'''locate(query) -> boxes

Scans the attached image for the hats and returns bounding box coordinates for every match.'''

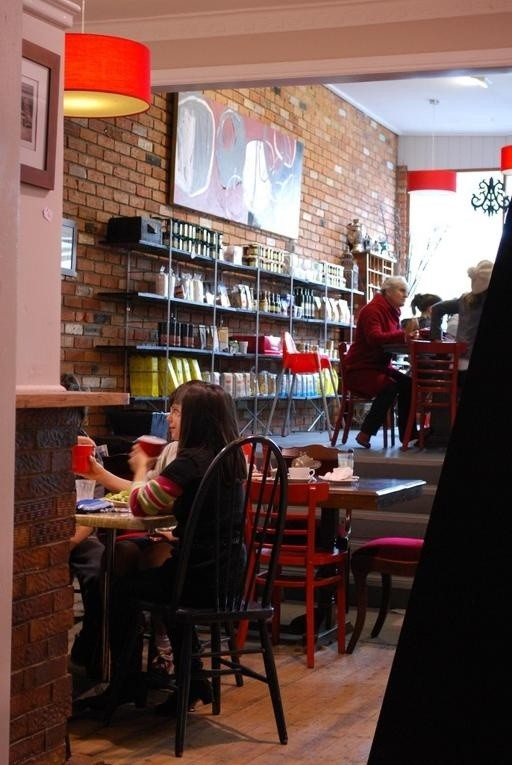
[467,260,493,294]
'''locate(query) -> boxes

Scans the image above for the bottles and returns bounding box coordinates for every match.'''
[155,266,168,296]
[157,320,167,346]
[181,324,189,347]
[303,289,308,316]
[163,217,224,261]
[259,290,267,311]
[310,289,315,320]
[242,242,287,274]
[265,290,282,314]
[176,323,181,346]
[168,322,176,347]
[295,287,305,319]
[187,325,195,347]
[168,269,175,297]
[305,289,311,320]
[291,254,351,288]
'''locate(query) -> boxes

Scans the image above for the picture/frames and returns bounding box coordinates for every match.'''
[20,39,61,190]
[61,216,77,276]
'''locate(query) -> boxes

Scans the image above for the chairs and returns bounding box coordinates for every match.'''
[116,434,289,758]
[233,475,349,669]
[403,341,461,451]
[242,436,272,475]
[346,537,425,654]
[329,342,396,449]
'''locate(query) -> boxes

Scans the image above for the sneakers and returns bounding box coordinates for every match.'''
[401,431,419,442]
[355,433,370,448]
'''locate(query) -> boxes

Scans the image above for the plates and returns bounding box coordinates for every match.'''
[102,497,132,508]
[319,475,358,483]
[286,479,313,483]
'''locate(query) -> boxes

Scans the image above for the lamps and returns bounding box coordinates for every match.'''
[407,99,456,194]
[501,145,511,172]
[64,0,150,118]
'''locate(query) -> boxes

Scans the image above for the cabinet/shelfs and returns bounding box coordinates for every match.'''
[93,214,365,438]
[343,251,395,342]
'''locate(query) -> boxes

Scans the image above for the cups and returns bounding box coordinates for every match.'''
[76,480,96,503]
[338,453,354,470]
[334,468,353,480]
[71,445,95,478]
[136,435,167,470]
[288,468,315,479]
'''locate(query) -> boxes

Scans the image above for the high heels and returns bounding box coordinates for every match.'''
[156,677,213,714]
[79,679,147,710]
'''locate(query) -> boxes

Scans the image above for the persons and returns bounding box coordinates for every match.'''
[429,260,493,384]
[76,379,204,674]
[446,297,470,384]
[339,275,431,449]
[402,293,442,390]
[73,383,248,711]
[60,372,109,670]
